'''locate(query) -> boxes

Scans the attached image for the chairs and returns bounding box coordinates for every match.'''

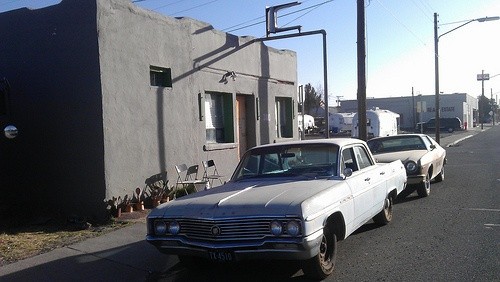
[185,165,208,192]
[173,164,194,199]
[202,161,224,187]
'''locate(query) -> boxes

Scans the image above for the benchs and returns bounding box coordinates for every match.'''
[283,163,336,175]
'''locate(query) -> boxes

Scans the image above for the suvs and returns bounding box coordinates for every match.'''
[416,116,463,133]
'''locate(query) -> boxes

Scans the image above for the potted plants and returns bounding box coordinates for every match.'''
[126,203,133,213]
[152,192,161,208]
[137,200,144,212]
[115,205,121,217]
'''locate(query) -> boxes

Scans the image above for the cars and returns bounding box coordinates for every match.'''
[146,137,408,282]
[344,133,448,198]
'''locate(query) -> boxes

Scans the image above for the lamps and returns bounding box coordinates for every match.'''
[222,71,237,83]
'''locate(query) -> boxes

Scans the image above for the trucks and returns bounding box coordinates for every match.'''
[297,112,315,135]
[350,106,400,141]
[329,111,356,134]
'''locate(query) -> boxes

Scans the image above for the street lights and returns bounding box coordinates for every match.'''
[433,16,500,146]
[481,73,500,130]
[418,94,423,134]
